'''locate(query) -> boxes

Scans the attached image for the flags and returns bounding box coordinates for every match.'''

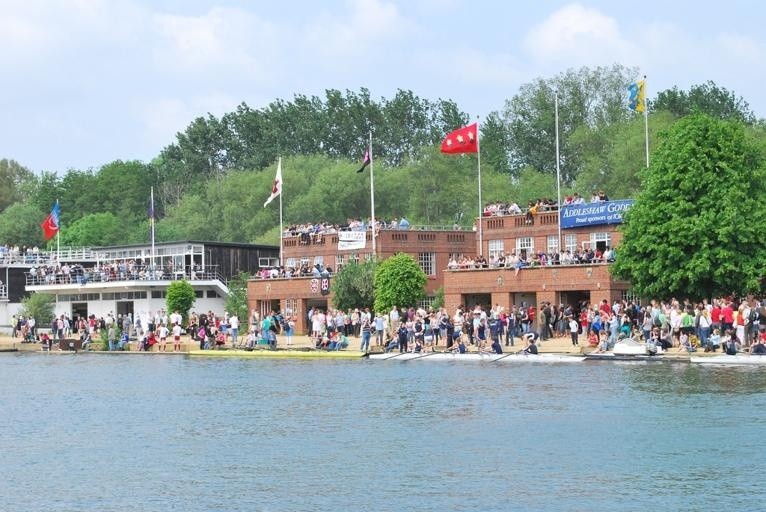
[41,204,60,241]
[441,122,479,157]
[261,162,284,207]
[355,143,373,175]
[147,199,157,241]
[628,76,647,114]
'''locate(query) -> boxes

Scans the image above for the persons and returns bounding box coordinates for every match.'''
[30,261,200,284]
[453,222,463,231]
[562,190,609,206]
[256,264,333,279]
[305,299,766,355]
[1,244,39,266]
[482,199,522,217]
[285,216,410,244]
[11,312,294,354]
[447,245,617,272]
[527,198,558,225]
